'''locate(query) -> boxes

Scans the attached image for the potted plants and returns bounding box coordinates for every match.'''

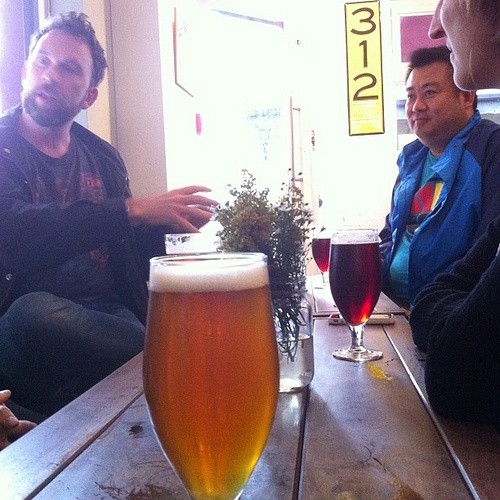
[213,169,327,394]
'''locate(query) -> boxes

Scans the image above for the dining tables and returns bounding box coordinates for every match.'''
[0,273,500,500]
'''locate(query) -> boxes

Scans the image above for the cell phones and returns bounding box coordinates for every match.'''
[328,313,395,325]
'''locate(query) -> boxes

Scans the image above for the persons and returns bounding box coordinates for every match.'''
[379,44,500,308]
[408,0,499,444]
[0,10,217,398]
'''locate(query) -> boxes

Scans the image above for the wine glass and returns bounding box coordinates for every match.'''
[140,251,280,500]
[328,229,383,362]
[311,231,331,289]
